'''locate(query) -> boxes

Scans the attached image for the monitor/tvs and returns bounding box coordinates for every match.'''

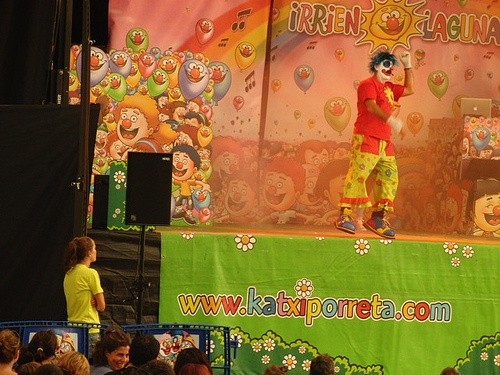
[460,97,492,120]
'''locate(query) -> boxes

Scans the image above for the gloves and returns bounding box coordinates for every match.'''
[398,52,412,68]
[387,115,403,134]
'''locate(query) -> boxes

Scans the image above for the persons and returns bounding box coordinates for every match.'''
[0,329,90,375]
[63,237,106,363]
[90,329,175,375]
[440,367,459,375]
[334,51,415,238]
[264,354,335,375]
[175,348,212,375]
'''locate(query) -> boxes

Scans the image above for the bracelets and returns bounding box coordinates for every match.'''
[404,68,410,70]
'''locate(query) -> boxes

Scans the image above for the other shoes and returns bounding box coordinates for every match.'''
[336,208,355,234]
[362,211,395,239]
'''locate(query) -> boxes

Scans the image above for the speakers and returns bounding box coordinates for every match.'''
[92,175,125,229]
[125,151,172,226]
